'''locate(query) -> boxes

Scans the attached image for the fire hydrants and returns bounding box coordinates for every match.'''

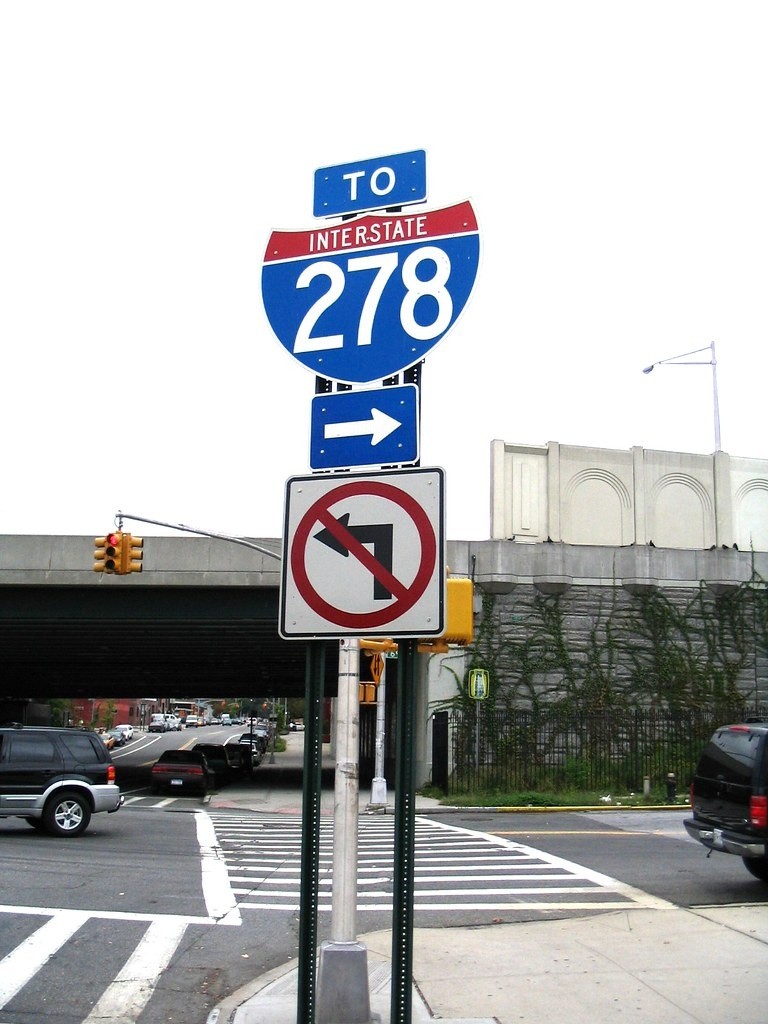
[665,772,677,804]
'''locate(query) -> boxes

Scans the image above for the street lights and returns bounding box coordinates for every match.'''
[644,339,724,453]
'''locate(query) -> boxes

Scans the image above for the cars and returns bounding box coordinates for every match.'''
[209,718,218,724]
[217,713,305,760]
[192,742,230,787]
[226,742,250,779]
[98,733,114,750]
[151,749,215,794]
[198,717,206,726]
[107,729,127,746]
[115,724,134,741]
[148,713,182,732]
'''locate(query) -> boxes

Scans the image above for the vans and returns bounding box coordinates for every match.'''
[185,715,199,728]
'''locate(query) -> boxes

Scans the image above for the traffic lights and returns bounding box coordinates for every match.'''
[105,530,124,574]
[94,535,105,573]
[125,533,144,574]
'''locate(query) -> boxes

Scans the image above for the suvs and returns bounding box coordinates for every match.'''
[682,722,768,885]
[0,722,127,839]
